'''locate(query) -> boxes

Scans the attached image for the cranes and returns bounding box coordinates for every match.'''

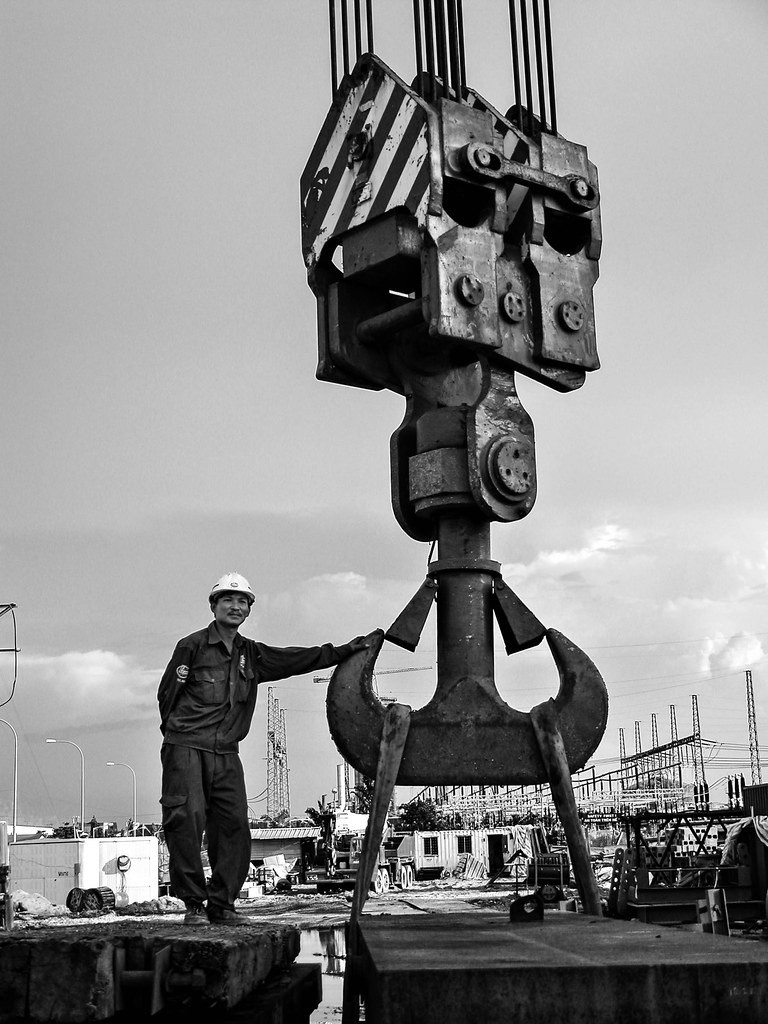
[312,661,434,815]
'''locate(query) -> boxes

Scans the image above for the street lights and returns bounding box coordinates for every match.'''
[105,761,137,837]
[44,738,85,836]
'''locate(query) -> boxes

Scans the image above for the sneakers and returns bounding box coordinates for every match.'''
[206,904,251,927]
[185,904,210,925]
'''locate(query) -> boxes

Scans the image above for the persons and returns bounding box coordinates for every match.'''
[149,567,382,929]
[85,815,98,838]
[106,818,133,837]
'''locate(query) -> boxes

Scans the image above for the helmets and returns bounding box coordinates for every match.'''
[208,572,255,607]
